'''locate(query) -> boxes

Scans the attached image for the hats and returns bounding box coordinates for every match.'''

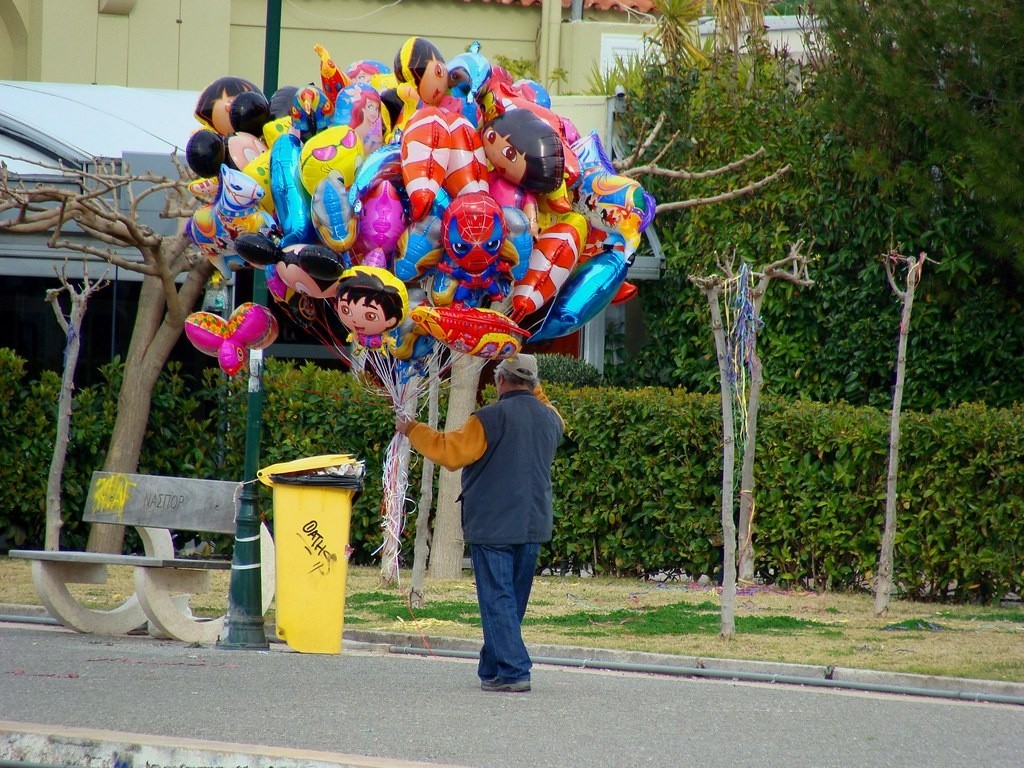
[499,353,538,381]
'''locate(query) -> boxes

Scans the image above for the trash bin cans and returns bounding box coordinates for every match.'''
[256,454,367,656]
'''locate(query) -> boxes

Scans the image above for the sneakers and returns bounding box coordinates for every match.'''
[481,674,531,692]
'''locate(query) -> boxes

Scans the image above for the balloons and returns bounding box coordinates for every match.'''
[172,38,652,373]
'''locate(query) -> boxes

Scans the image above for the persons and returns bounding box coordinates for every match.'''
[395,353,565,690]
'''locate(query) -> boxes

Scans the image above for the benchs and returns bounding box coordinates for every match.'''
[9,473,276,651]
